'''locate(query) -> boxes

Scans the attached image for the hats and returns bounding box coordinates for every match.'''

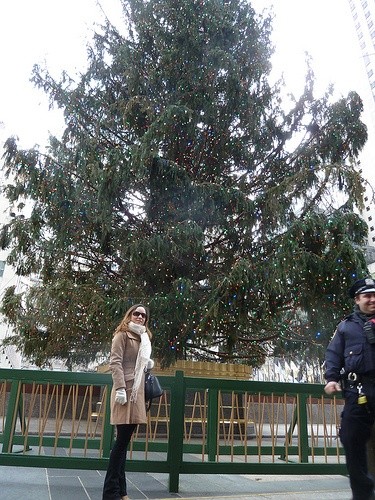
[350,278,375,299]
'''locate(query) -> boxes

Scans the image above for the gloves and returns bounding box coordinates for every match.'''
[115,387,127,405]
[147,359,154,369]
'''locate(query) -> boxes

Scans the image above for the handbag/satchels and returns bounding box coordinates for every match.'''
[144,374,164,401]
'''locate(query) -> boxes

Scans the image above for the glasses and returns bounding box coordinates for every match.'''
[133,311,146,318]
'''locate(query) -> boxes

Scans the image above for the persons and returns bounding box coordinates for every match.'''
[102,304,154,500]
[324,279,375,500]
